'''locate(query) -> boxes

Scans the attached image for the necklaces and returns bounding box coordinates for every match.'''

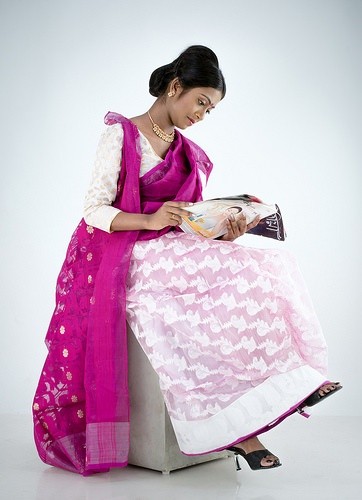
[147,110,177,143]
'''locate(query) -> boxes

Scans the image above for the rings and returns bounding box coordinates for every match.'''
[170,214,176,219]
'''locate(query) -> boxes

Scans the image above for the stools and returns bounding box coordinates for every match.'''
[124,318,237,474]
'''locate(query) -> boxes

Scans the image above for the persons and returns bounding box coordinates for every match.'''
[190,206,242,236]
[62,43,344,472]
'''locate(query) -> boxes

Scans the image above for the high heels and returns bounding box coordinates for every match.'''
[296,382,343,418]
[226,447,282,470]
